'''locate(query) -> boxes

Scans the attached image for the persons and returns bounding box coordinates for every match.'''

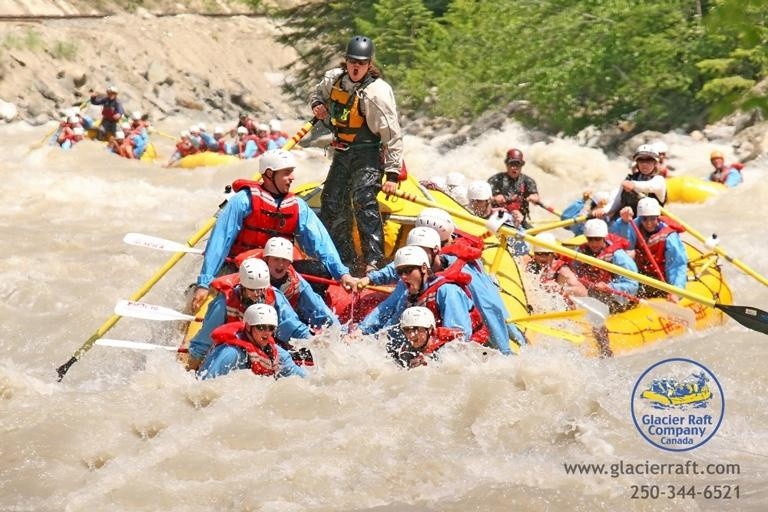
[51,86,153,160]
[187,137,690,379]
[174,111,289,158]
[306,35,403,273]
[705,150,745,187]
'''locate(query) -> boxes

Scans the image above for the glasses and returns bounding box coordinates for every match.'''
[403,326,418,333]
[587,237,603,241]
[349,58,368,65]
[396,266,415,275]
[255,325,275,331]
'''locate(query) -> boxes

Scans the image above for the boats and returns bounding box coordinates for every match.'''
[509,231,731,363]
[173,168,529,381]
[655,173,732,205]
[166,148,250,171]
[78,113,155,167]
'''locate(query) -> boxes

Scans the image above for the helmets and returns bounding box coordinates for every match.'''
[400,306,437,331]
[239,111,250,119]
[239,236,293,326]
[58,107,83,136]
[593,190,610,207]
[632,139,668,163]
[346,35,374,60]
[585,218,608,238]
[258,148,298,173]
[107,87,118,94]
[711,150,724,160]
[533,232,555,253]
[446,172,465,187]
[237,119,281,135]
[468,181,492,201]
[637,197,661,217]
[394,207,454,269]
[505,149,525,166]
[115,111,142,139]
[180,123,225,137]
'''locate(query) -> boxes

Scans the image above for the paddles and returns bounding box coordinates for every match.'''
[370,182,768,336]
[115,299,206,322]
[125,233,354,292]
[577,277,697,327]
[56,117,320,389]
[93,338,193,353]
[507,295,612,326]
